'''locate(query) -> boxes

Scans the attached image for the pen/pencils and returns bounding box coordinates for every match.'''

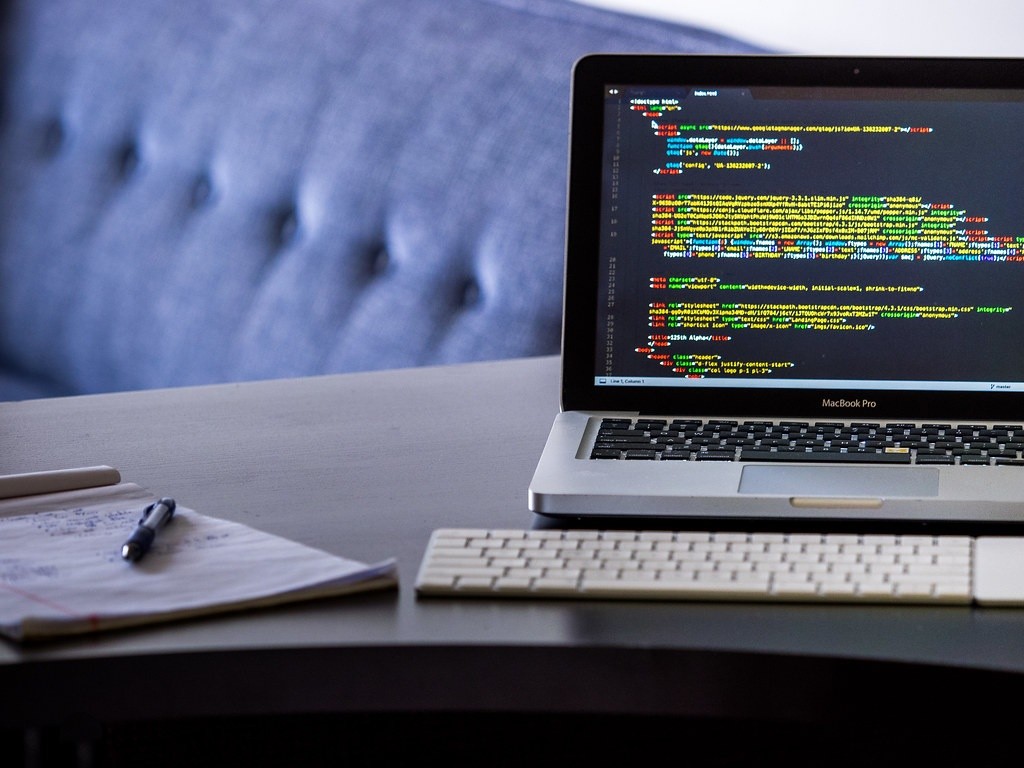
[120,497,179,568]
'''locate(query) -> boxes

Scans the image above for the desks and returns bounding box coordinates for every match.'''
[0,353,1022,766]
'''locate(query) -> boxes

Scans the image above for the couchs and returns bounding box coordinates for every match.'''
[0,1,788,399]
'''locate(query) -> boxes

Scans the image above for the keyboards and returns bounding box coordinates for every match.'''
[413,528,972,600]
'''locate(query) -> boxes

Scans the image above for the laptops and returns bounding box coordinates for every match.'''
[524,53,1024,522]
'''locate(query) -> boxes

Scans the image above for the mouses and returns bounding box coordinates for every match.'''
[977,539,1024,604]
